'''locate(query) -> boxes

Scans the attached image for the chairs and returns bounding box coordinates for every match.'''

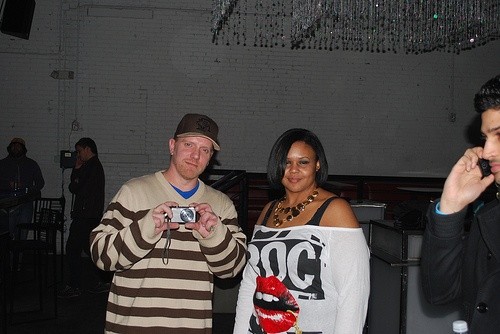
[7,196,65,325]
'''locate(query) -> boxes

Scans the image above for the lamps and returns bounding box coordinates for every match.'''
[207,0,500,54]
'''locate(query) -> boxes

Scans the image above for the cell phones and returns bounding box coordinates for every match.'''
[479,159,491,176]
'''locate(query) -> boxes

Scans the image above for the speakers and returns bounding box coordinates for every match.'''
[0,0,36,41]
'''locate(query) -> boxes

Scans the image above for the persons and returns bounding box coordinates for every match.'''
[89,113,248,334]
[418,73,499,333]
[231,127,371,334]
[57,137,111,298]
[0,137,45,271]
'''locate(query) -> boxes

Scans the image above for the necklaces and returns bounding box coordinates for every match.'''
[273,189,319,227]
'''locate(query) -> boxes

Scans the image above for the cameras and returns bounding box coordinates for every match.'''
[164,207,196,223]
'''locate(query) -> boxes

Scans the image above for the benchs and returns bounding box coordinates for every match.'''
[196,168,500,231]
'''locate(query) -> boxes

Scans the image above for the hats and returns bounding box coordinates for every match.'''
[174,113,220,151]
[7,138,27,153]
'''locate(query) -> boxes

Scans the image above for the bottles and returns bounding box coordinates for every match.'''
[453,320,470,334]
